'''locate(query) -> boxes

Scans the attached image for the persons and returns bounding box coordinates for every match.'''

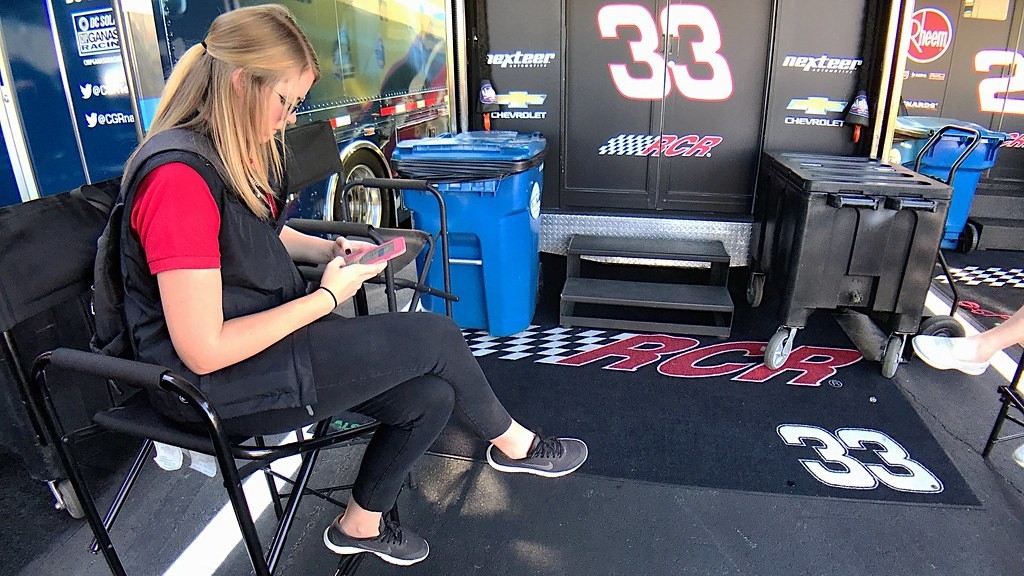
[117,4,587,565]
[911,305,1024,469]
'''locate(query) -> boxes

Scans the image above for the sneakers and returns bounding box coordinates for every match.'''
[323,514,430,567]
[486,432,588,478]
[912,335,990,375]
[1013,444,1024,467]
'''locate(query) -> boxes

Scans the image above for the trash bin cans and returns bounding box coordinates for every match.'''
[758,146,952,331]
[389,131,549,337]
[889,115,1006,252]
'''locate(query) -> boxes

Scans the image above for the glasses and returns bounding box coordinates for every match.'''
[254,77,306,115]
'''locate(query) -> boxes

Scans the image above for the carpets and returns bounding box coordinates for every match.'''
[301,306,988,515]
[930,247,1024,368]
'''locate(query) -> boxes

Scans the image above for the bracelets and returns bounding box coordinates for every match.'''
[319,287,337,309]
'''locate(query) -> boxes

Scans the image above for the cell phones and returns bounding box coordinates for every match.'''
[340,237,406,269]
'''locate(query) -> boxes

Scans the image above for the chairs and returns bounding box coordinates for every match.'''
[0,173,403,576]
[268,117,461,321]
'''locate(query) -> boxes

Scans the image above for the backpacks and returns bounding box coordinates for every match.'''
[70,129,234,398]
